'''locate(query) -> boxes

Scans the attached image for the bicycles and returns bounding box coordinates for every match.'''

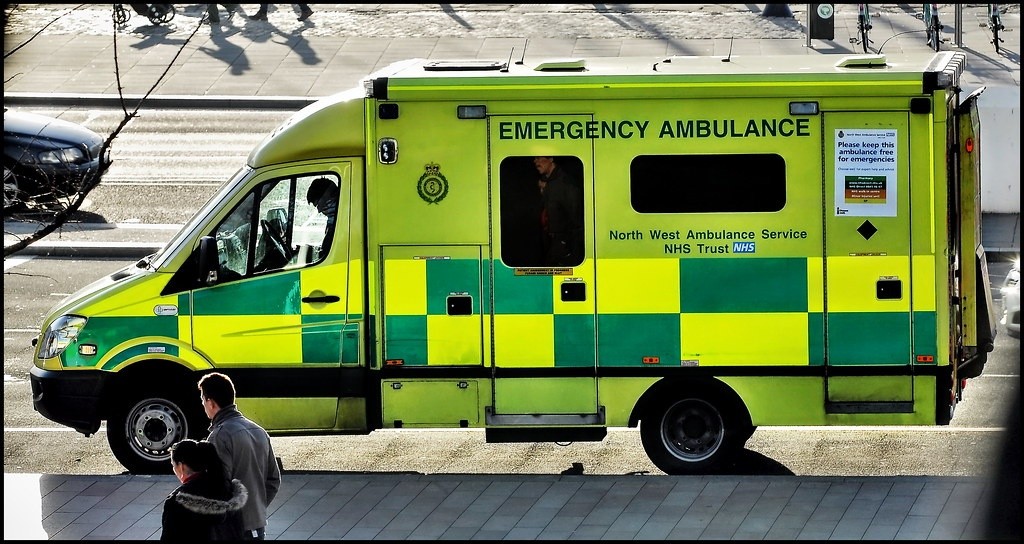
[849,4,880,52]
[915,3,944,51]
[985,4,1005,53]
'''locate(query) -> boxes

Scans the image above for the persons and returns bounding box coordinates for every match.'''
[307,177,338,257]
[159,439,247,539]
[197,373,281,539]
[501,156,585,266]
[199,4,315,24]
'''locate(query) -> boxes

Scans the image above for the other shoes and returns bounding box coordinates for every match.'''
[249,10,268,21]
[299,8,314,21]
[203,17,219,25]
[227,7,237,22]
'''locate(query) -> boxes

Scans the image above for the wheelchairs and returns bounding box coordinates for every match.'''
[111,3,175,25]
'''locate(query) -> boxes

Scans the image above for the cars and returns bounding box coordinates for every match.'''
[4,107,110,216]
[1002,260,1020,340]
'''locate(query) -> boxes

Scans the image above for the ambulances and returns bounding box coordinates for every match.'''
[29,52,998,475]
